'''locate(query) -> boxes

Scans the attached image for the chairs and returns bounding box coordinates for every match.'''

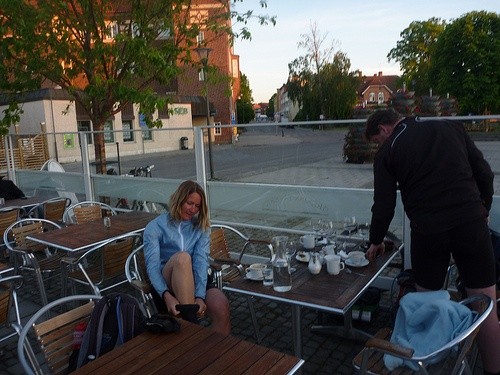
[0,195,493,375]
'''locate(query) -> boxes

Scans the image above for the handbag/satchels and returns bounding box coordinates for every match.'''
[383,289,480,371]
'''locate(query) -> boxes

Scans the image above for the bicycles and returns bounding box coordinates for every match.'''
[110,165,169,214]
[339,224,458,290]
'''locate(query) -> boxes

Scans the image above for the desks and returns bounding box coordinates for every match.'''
[25,210,161,308]
[72,316,306,375]
[221,234,405,375]
[1,193,63,228]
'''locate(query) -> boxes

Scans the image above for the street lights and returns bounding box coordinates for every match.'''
[195,46,218,181]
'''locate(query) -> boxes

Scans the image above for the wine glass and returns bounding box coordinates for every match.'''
[313,225,322,246]
[358,223,369,250]
[343,217,357,247]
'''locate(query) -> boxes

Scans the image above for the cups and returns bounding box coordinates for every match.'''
[318,218,335,236]
[325,255,345,275]
[262,268,274,287]
[348,251,365,264]
[299,234,315,249]
[245,263,267,277]
[104,217,111,227]
[336,232,346,255]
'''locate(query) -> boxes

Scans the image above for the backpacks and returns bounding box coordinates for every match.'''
[74,292,147,371]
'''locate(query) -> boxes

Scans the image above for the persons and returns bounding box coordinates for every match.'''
[143,180,230,335]
[365,109,500,375]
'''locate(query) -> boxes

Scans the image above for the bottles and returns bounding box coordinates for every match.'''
[308,256,322,275]
[272,236,293,293]
[72,322,87,349]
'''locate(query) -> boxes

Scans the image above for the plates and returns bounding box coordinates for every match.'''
[344,259,369,267]
[295,254,310,262]
[245,272,263,280]
